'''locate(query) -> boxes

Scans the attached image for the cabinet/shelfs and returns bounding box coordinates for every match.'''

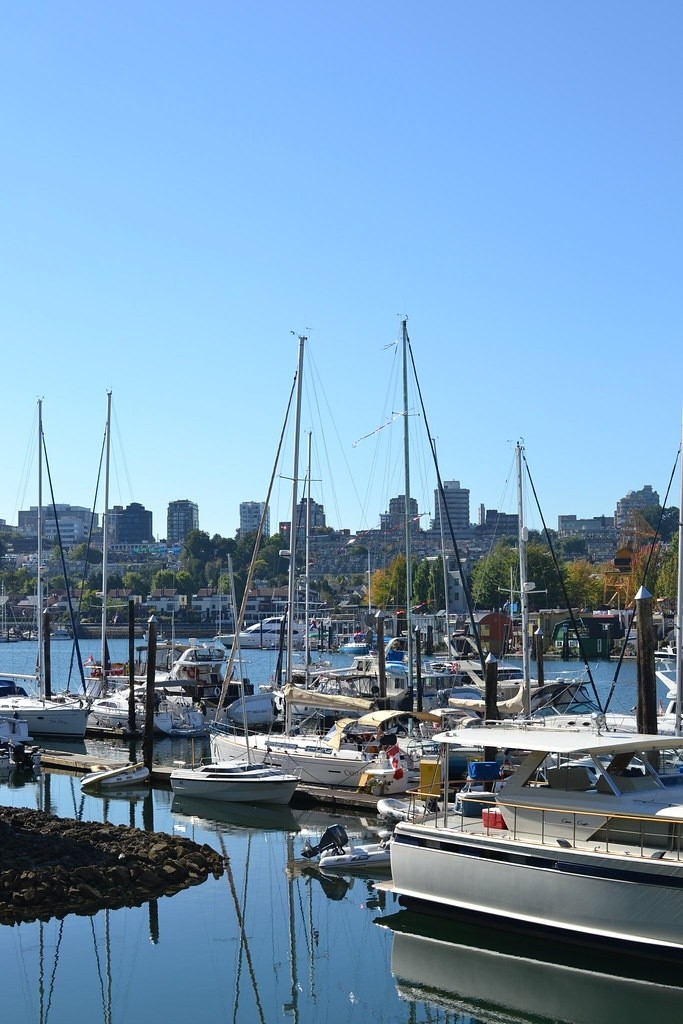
[419,760,442,800]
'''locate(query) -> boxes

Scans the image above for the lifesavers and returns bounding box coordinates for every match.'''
[213,688,221,697]
[451,663,461,673]
[186,667,197,677]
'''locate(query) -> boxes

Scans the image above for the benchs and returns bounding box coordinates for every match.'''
[545,768,595,791]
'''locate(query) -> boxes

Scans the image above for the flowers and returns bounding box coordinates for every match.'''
[367,777,392,791]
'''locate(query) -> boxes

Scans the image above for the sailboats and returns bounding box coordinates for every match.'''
[0,312,683,955]
[26,738,88,820]
[168,794,304,1024]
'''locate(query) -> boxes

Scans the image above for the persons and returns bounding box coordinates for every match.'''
[154,693,207,717]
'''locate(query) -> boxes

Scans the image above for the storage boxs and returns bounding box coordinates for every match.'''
[455,791,498,816]
[482,808,506,829]
[468,762,499,780]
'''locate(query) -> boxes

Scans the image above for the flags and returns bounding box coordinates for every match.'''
[309,617,317,632]
[388,745,403,779]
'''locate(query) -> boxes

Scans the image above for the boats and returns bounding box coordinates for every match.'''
[319,867,390,883]
[372,908,683,1024]
[80,784,150,798]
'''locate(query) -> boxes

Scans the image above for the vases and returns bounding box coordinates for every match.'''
[371,786,383,796]
